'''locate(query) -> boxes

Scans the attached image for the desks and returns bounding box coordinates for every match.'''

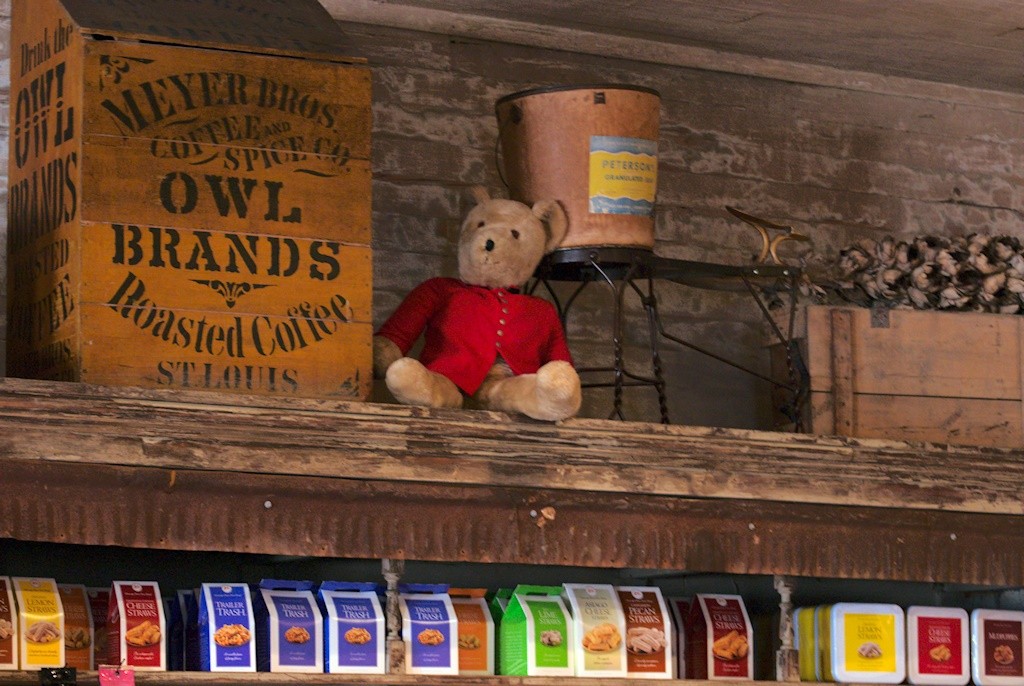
[522,250,805,431]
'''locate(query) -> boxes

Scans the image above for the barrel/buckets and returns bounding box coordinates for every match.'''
[492,83,659,251]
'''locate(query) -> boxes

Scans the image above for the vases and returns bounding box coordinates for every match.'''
[806,305,1024,447]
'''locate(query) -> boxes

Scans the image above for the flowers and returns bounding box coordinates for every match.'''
[821,230,1024,310]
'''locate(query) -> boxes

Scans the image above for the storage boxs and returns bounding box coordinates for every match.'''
[10,0,373,399]
[793,604,1024,686]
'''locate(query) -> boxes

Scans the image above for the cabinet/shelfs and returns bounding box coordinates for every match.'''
[0,374,1024,686]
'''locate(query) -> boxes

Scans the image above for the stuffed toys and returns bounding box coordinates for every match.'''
[372,184,582,422]
